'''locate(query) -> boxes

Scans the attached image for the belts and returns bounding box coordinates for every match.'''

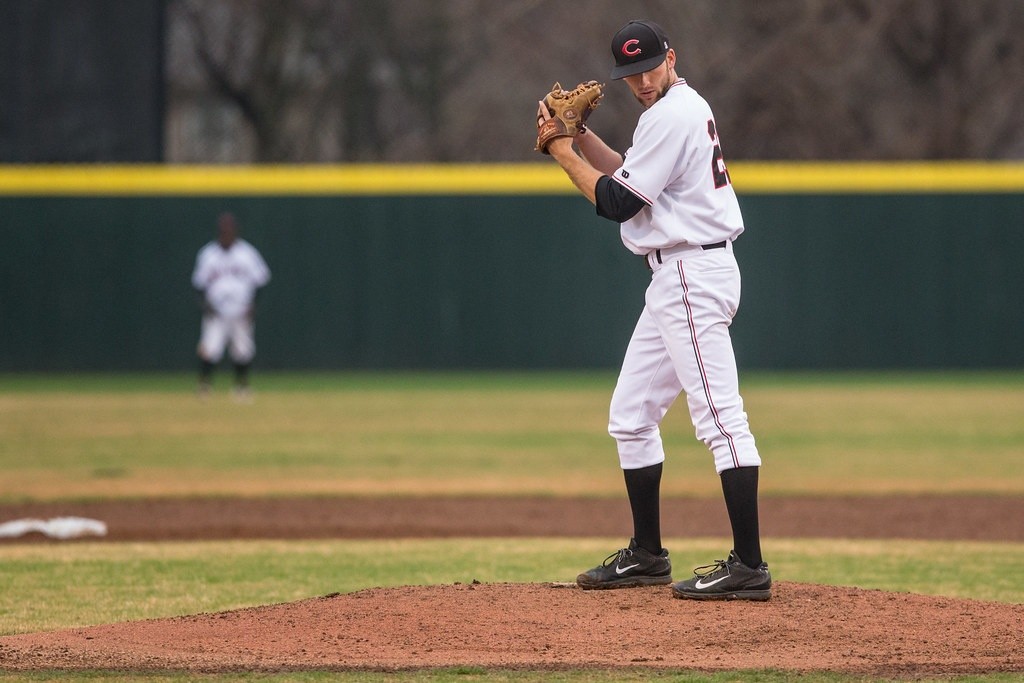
[644,240,726,270]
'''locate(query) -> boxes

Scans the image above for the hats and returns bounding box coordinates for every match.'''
[609,20,671,81]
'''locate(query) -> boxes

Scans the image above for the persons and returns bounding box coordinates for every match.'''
[192,213,271,383]
[534,20,773,601]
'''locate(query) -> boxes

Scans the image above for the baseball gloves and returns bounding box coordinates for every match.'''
[534,79,606,155]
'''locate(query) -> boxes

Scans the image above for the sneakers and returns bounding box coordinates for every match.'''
[672,550,771,601]
[576,537,674,590]
